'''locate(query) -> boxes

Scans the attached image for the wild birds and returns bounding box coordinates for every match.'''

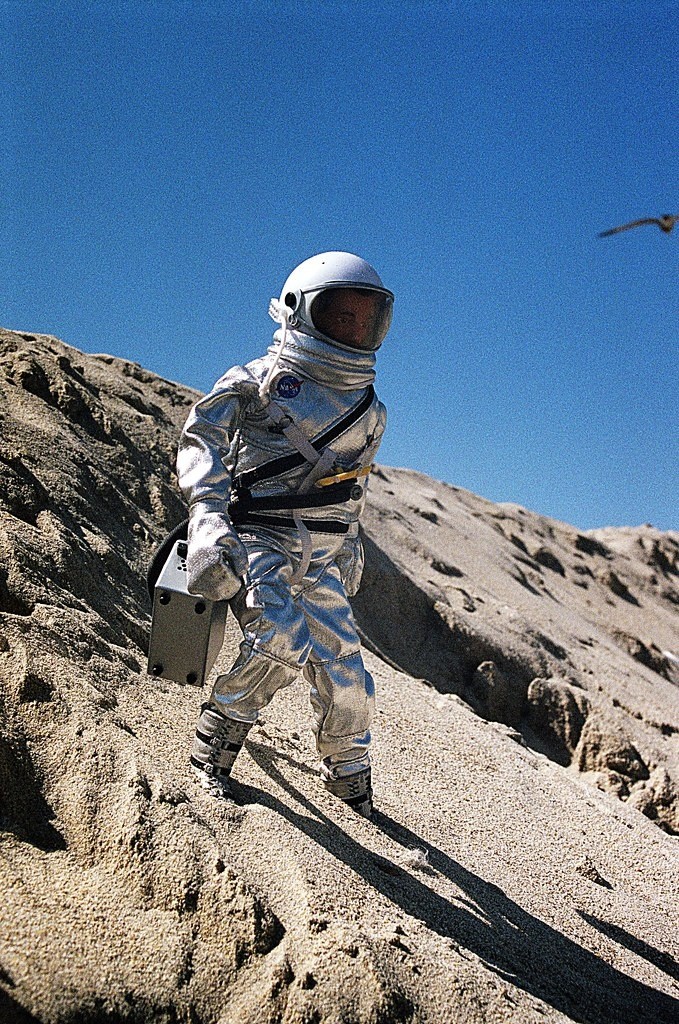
[599,214,679,238]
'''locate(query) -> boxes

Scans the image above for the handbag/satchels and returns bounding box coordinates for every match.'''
[145,518,229,688]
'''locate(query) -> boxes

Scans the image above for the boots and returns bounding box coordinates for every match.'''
[319,765,372,822]
[191,702,254,803]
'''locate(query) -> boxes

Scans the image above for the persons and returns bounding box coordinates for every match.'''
[177,249,396,816]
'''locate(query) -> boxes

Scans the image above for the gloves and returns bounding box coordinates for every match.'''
[185,496,249,603]
[335,521,364,598]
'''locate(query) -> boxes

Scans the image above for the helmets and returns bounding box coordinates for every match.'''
[269,250,396,356]
